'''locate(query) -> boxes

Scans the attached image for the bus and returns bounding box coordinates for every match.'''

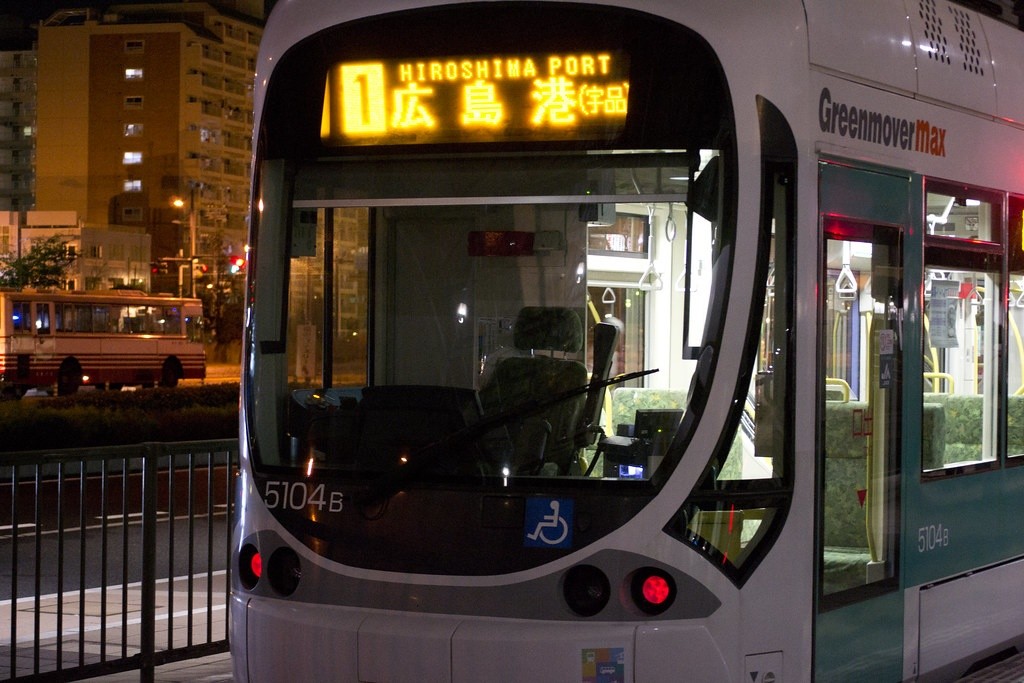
[0,289,209,402]
[226,0,1023,683]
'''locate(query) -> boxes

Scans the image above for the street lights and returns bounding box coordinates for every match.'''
[170,182,203,300]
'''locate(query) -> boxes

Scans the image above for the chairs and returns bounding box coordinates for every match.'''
[437,303,1024,594]
